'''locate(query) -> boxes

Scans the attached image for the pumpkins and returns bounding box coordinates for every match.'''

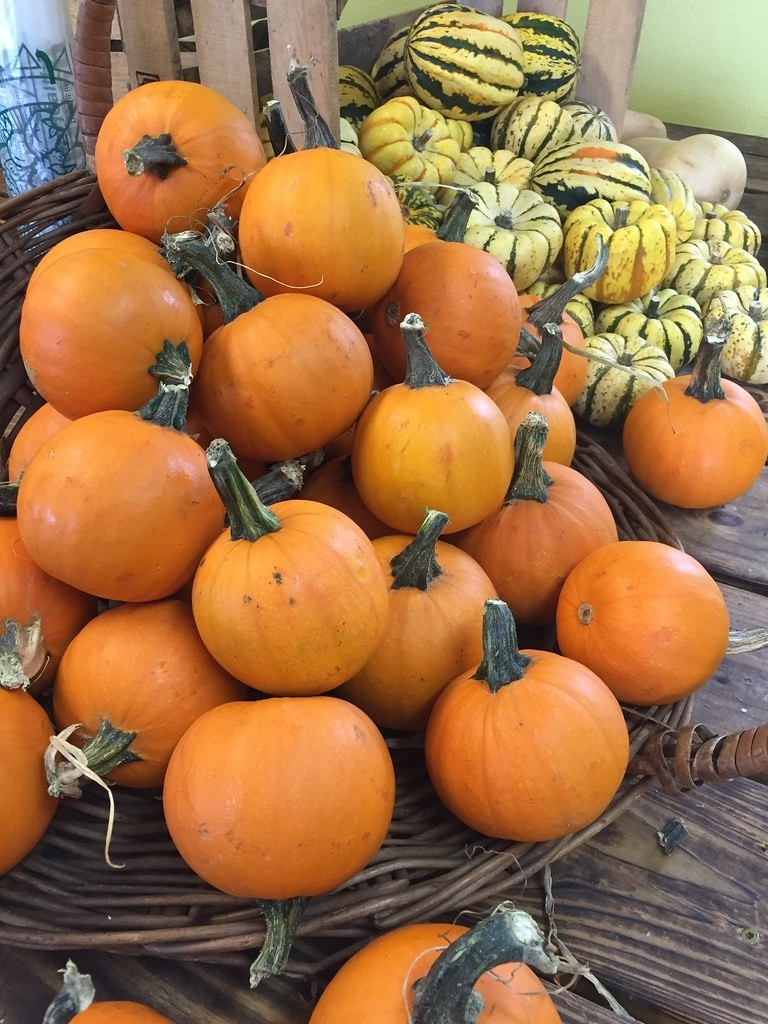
[0,4,768,1024]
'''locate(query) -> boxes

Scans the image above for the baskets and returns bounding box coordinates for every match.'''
[0,0,768,976]
